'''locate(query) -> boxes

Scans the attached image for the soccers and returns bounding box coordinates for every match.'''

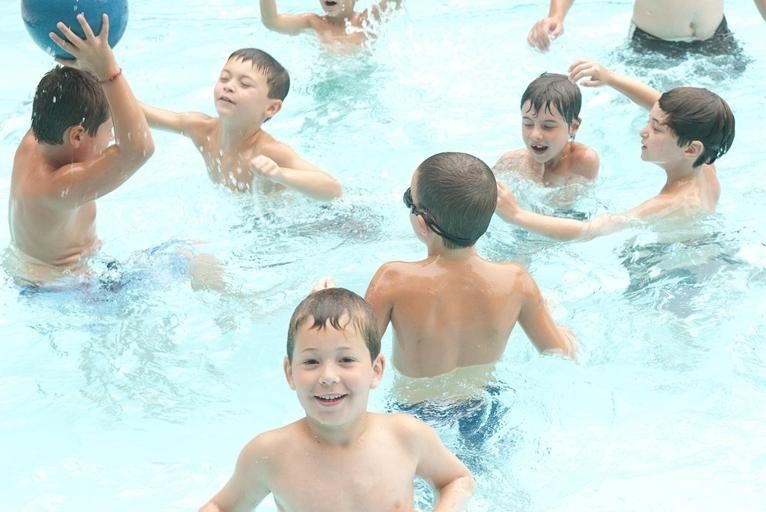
[21,0,128,61]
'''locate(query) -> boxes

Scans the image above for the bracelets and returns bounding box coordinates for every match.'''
[99,68,122,83]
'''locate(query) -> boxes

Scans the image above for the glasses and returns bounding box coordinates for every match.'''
[403,188,472,246]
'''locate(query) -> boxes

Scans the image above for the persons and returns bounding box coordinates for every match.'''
[260,0,403,57]
[310,152,579,473]
[4,14,221,309]
[527,0,766,86]
[495,59,735,243]
[198,288,476,512]
[491,73,599,208]
[137,48,342,202]
